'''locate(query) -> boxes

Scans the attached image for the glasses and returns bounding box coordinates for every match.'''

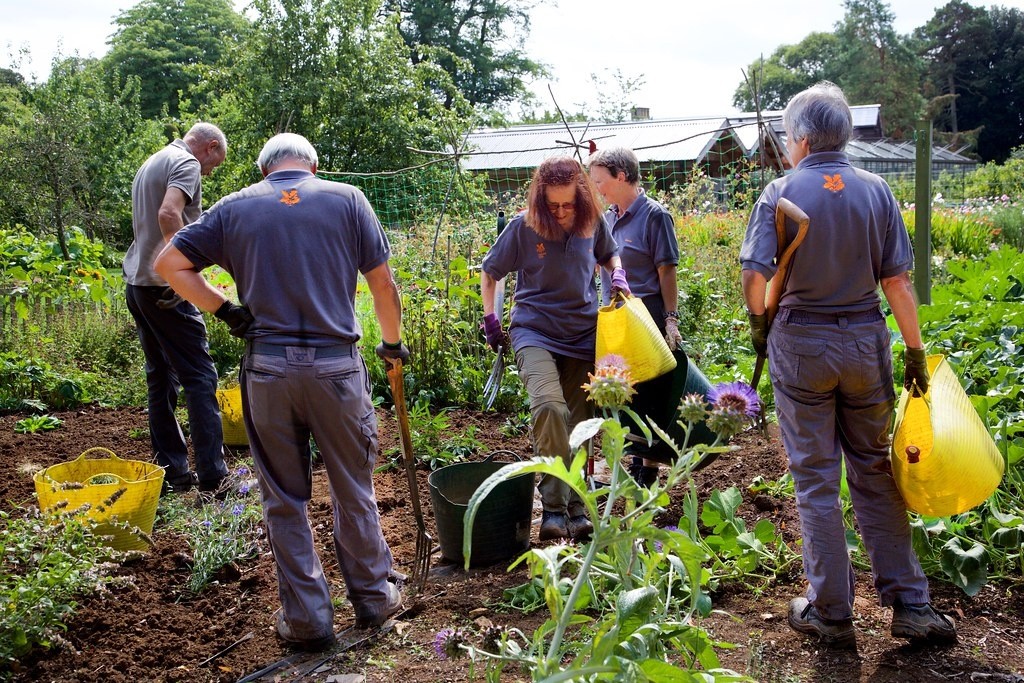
[543,198,577,212]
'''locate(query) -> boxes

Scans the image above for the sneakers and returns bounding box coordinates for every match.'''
[628,464,657,488]
[538,510,570,538]
[786,596,855,640]
[892,601,956,637]
[567,515,593,537]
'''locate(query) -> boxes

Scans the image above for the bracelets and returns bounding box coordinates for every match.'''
[664,312,679,322]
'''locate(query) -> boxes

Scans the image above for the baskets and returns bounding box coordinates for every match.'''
[427,450,536,569]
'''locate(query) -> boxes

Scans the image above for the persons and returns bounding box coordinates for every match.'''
[481,155,631,540]
[123,122,250,505]
[154,132,409,642]
[739,84,955,645]
[587,147,682,490]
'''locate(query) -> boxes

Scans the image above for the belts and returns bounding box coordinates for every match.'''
[251,342,352,358]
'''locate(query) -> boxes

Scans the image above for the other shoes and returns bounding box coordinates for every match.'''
[199,486,234,502]
[278,613,336,646]
[161,470,198,493]
[358,580,402,627]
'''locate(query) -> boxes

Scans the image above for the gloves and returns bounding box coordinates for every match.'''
[903,344,930,398]
[482,312,509,353]
[663,316,682,350]
[375,339,409,370]
[214,302,254,337]
[156,284,186,309]
[748,309,769,358]
[608,268,632,301]
